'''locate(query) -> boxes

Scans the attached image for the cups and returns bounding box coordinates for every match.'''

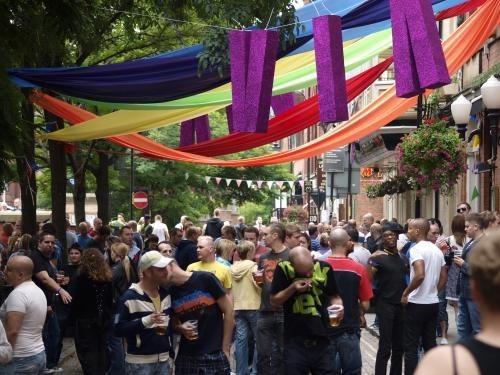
[156,313,169,336]
[56,271,64,283]
[254,271,263,283]
[327,305,344,327]
[185,320,198,340]
[454,251,462,259]
[437,235,450,254]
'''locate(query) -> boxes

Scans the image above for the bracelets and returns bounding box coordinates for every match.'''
[56,287,63,293]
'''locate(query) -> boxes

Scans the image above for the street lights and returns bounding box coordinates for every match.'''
[481,75,499,211]
[451,94,471,208]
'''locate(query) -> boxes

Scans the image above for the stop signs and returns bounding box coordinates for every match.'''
[132,192,149,210]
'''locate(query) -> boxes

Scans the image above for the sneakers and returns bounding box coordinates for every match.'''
[440,338,448,345]
[369,323,380,336]
[47,367,63,373]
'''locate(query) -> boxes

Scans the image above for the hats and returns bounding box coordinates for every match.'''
[140,250,174,273]
[382,225,405,234]
[144,215,150,219]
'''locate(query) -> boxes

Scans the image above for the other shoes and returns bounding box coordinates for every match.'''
[438,332,443,337]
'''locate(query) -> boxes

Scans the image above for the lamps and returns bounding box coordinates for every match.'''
[481,74,500,146]
[449,94,484,151]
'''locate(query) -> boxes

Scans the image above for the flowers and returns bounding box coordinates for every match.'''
[395,115,476,200]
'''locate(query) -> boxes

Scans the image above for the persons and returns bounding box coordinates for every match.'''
[0,197,500,375]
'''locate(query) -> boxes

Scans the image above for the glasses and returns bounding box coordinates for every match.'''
[429,220,439,225]
[457,208,467,212]
